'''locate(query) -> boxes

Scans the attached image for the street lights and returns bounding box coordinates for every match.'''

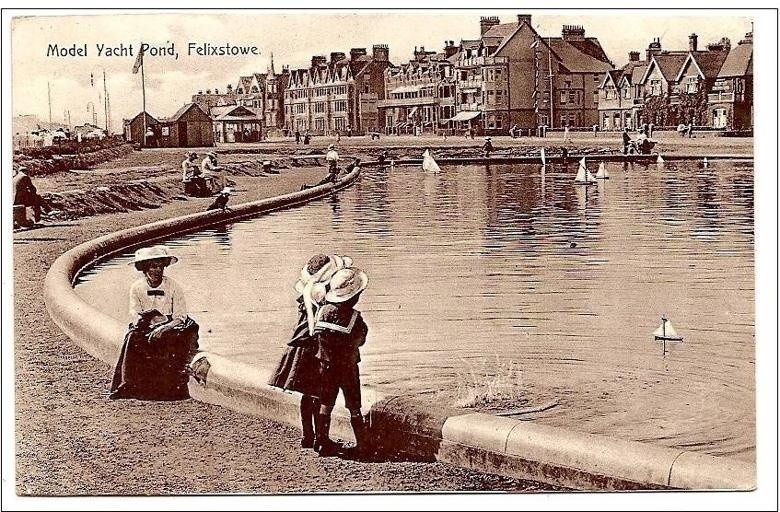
[536,23,555,135]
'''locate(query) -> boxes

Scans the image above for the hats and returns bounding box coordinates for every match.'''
[127,246,178,265]
[295,255,368,305]
[19,166,28,171]
[221,187,231,195]
[327,144,335,151]
[207,151,217,160]
[184,151,199,160]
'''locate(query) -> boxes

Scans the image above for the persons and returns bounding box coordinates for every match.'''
[15,128,70,147]
[108,244,199,402]
[13,166,60,225]
[635,129,648,146]
[225,128,235,142]
[677,120,692,137]
[342,158,361,174]
[564,124,574,143]
[375,153,385,167]
[269,254,368,454]
[442,131,448,142]
[465,125,481,139]
[182,151,224,196]
[300,168,342,191]
[638,121,655,138]
[347,126,352,137]
[304,129,311,144]
[593,123,599,137]
[623,128,631,154]
[336,132,340,143]
[145,127,154,148]
[206,187,232,210]
[295,129,300,144]
[326,144,339,173]
[242,127,249,142]
[482,137,494,157]
[509,124,518,138]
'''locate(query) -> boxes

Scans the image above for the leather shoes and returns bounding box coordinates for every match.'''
[48,210,60,216]
[301,434,374,458]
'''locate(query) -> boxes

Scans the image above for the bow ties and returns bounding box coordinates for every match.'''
[147,290,164,296]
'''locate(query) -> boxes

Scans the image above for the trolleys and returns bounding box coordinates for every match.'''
[627,138,659,157]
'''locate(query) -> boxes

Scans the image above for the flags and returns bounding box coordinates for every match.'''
[132,45,142,74]
